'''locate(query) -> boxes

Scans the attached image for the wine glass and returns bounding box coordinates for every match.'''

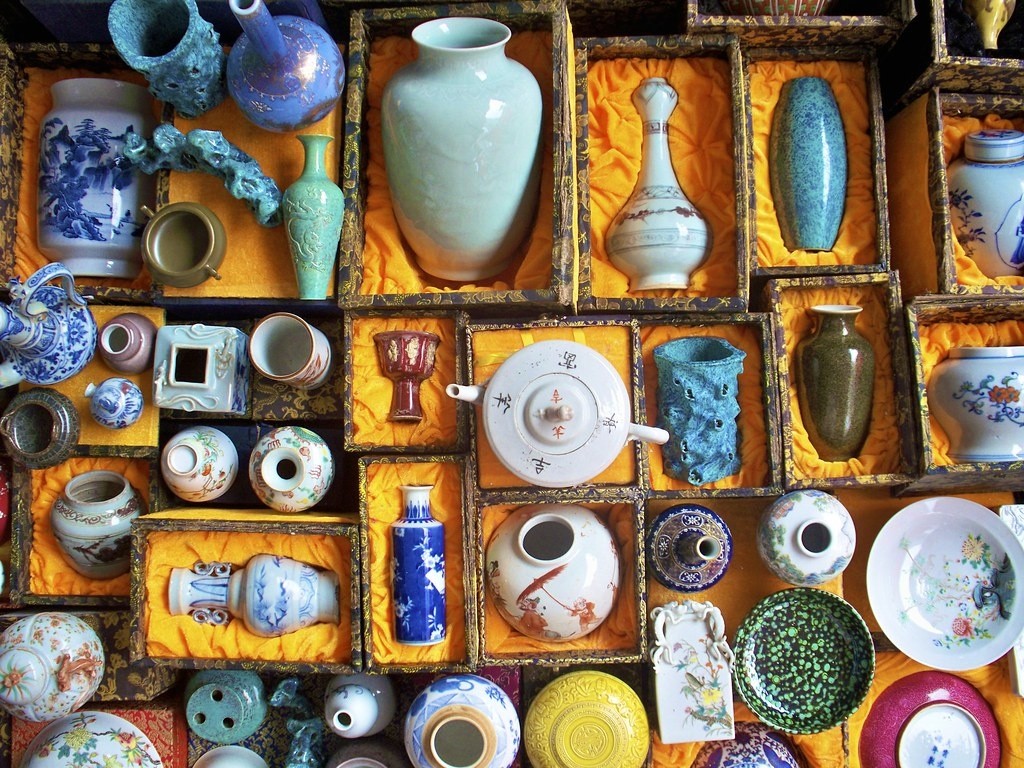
[373,331,440,421]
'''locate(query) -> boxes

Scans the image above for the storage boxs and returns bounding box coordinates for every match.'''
[0,0,1024,768]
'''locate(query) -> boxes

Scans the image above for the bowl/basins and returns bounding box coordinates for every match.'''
[141,202,227,287]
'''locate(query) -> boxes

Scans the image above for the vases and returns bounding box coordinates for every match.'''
[604,78,712,292]
[404,674,521,768]
[168,554,340,638]
[485,503,622,643]
[389,484,446,646]
[644,503,733,595]
[37,78,150,280]
[383,16,544,281]
[755,489,857,587]
[107,0,230,118]
[152,324,250,415]
[227,0,345,134]
[248,312,337,391]
[282,134,345,301]
[653,335,747,485]
[48,470,145,581]
[98,312,156,374]
[794,303,875,462]
[767,75,849,253]
[324,666,396,739]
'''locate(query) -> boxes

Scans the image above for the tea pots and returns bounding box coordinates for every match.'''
[446,339,669,489]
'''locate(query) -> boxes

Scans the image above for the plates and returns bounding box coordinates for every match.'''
[866,496,1024,671]
[732,587,875,735]
[858,670,1003,768]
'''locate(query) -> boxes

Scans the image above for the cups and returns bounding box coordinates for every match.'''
[249,312,338,390]
